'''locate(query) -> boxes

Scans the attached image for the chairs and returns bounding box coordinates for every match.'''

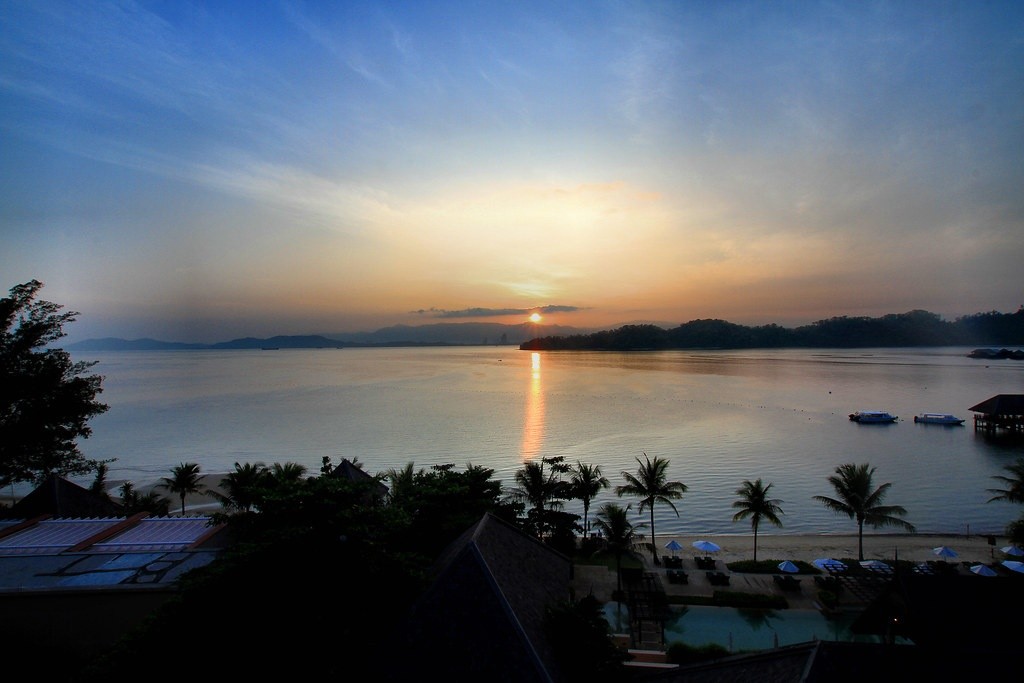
[785,576,801,591]
[672,556,683,569]
[677,571,688,584]
[826,576,840,591]
[667,570,680,583]
[694,557,707,569]
[707,572,722,585]
[718,572,731,585]
[705,557,716,570]
[814,576,832,590]
[773,576,792,591]
[663,556,674,568]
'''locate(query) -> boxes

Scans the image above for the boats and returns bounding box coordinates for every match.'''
[848,410,898,423]
[914,413,965,425]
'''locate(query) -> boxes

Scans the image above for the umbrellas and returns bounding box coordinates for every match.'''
[1000,546,1024,556]
[970,564,999,577]
[812,558,848,573]
[1001,560,1024,573]
[777,561,800,572]
[859,560,894,572]
[933,546,959,558]
[912,561,936,575]
[664,541,682,550]
[692,541,721,552]
